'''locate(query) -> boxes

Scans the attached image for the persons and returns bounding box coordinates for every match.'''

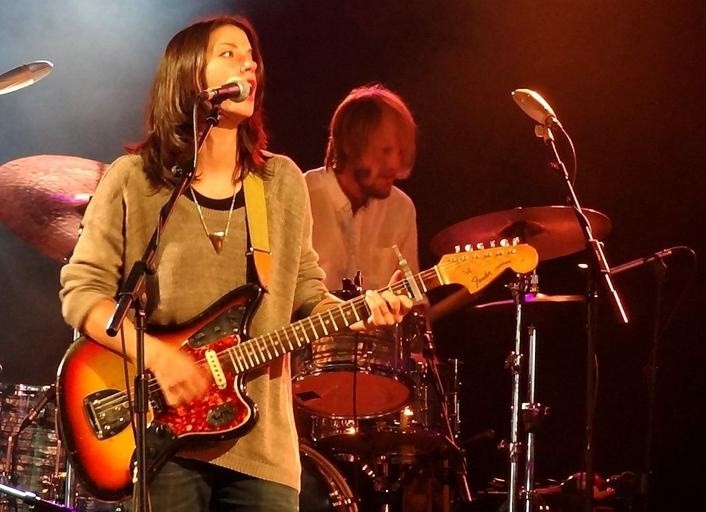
[300,86,423,298]
[65,29,415,512]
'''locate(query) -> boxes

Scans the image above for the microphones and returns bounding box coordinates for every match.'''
[511,89,562,134]
[1,59,53,97]
[20,386,56,431]
[196,76,250,102]
[600,245,687,276]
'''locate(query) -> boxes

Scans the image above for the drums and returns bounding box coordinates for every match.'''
[1,382,125,511]
[290,272,417,420]
[299,436,382,512]
[311,358,440,462]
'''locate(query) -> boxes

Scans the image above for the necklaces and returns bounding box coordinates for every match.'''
[187,176,239,255]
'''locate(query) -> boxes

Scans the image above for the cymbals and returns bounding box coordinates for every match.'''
[0,154,111,265]
[428,205,611,263]
[475,293,588,309]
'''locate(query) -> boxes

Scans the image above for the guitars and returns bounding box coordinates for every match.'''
[56,235,539,503]
